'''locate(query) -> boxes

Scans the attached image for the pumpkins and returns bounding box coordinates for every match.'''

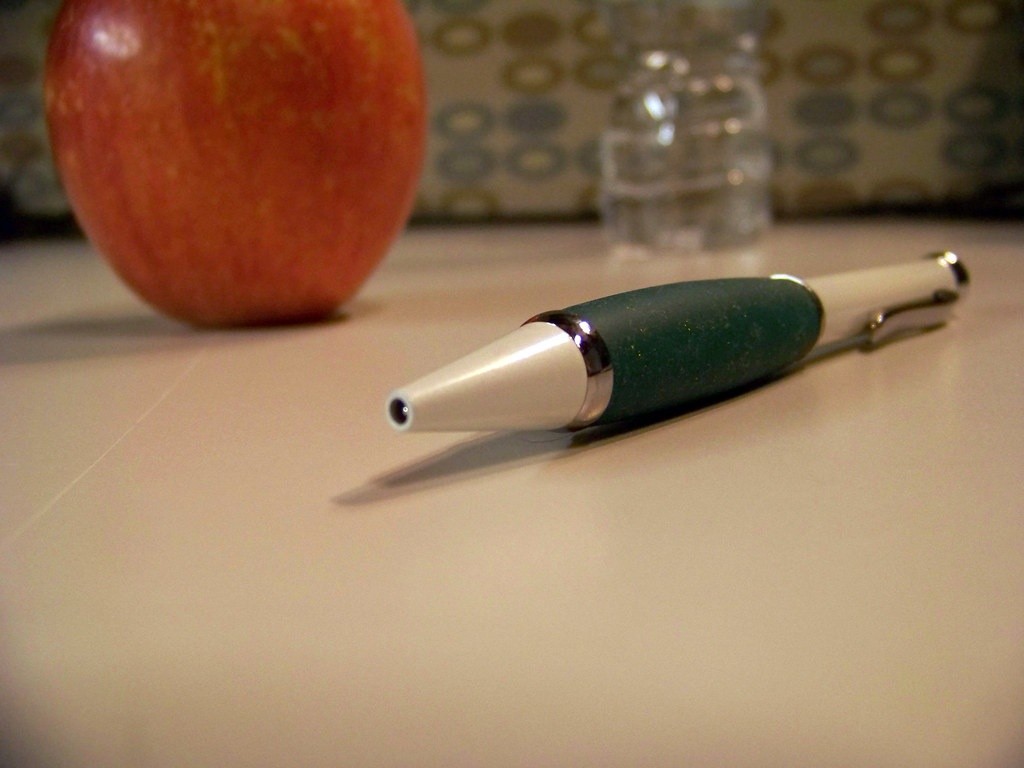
[43,0,428,327]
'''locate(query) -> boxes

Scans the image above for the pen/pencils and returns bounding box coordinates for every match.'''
[382,249,972,435]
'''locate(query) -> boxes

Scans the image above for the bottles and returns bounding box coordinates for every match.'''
[591,0,773,280]
